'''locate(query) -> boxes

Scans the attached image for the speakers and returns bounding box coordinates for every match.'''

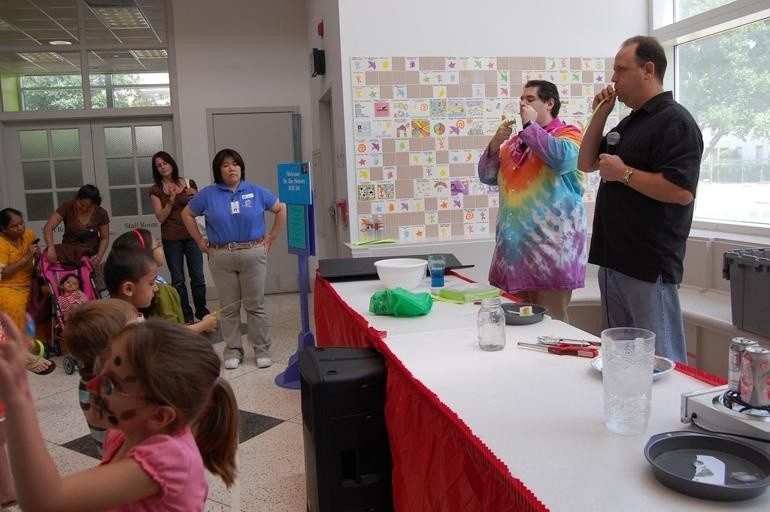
[297,346,395,512]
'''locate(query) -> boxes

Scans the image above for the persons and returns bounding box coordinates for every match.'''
[1,182,111,376]
[477,79,588,328]
[105,228,218,334]
[576,36,704,368]
[0,299,241,512]
[180,149,287,369]
[147,150,211,325]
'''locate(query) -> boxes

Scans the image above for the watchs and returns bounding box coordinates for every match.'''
[522,120,534,129]
[622,167,635,187]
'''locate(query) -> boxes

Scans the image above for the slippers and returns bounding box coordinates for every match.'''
[24,353,55,375]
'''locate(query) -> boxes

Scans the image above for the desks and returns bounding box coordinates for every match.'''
[312,263,770,511]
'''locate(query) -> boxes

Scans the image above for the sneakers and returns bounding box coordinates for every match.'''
[224,358,241,369]
[256,357,272,368]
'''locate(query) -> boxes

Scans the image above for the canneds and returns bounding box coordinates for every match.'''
[740,346,770,407]
[728,336,758,393]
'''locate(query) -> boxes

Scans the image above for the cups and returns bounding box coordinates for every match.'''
[428,255,446,288]
[600,328,657,437]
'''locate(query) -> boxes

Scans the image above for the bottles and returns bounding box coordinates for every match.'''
[360,215,384,242]
[477,298,506,352]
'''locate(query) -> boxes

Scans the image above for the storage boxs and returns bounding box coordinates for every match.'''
[722,249,769,340]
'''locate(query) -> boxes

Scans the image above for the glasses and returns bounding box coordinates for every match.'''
[93,357,164,403]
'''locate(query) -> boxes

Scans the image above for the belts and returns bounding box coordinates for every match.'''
[209,240,265,250]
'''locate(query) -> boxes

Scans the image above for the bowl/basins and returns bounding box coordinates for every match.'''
[374,258,428,291]
